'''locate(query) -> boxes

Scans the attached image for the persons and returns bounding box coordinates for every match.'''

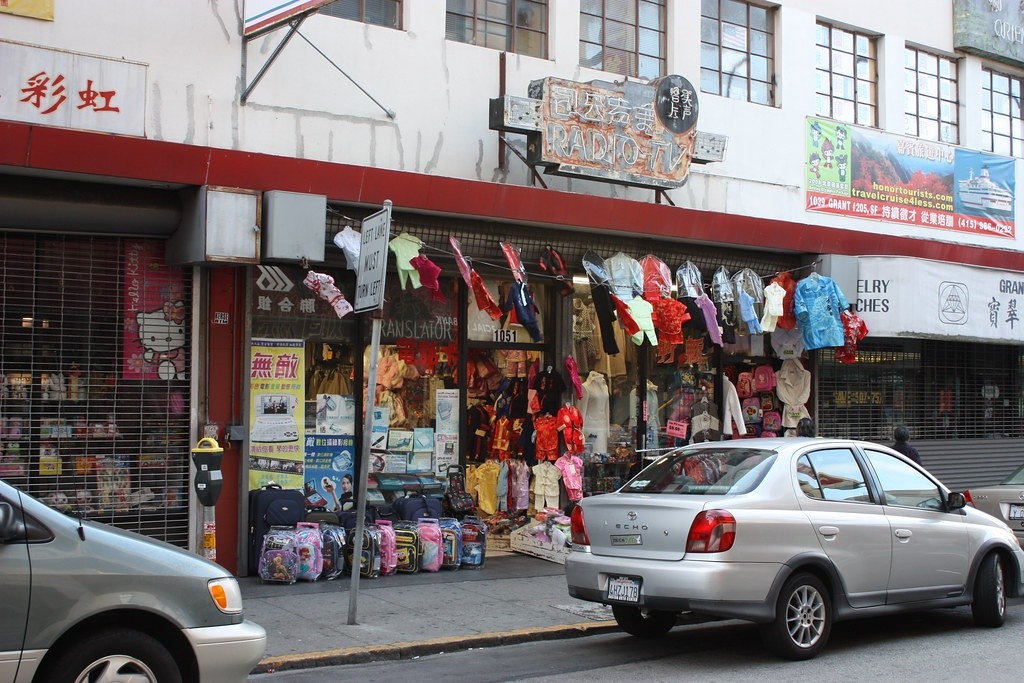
[253,460,302,472]
[305,482,315,493]
[577,371,610,452]
[795,418,816,438]
[266,399,273,407]
[324,474,354,512]
[889,426,921,467]
[279,399,284,407]
[628,378,661,456]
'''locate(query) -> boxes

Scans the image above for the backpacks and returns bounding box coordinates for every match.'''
[737,363,781,438]
[259,515,487,585]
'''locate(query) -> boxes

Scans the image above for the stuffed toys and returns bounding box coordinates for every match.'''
[0,373,119,513]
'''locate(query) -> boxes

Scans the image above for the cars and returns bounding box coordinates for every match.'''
[0,473,268,683]
[565,438,1024,651]
[962,461,1024,542]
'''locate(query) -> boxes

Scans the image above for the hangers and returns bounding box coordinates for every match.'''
[810,261,818,279]
[547,364,553,374]
[702,411,710,422]
[305,263,313,276]
[700,395,709,405]
[546,409,551,418]
[565,400,571,409]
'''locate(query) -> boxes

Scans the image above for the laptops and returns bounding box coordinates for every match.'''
[304,480,323,504]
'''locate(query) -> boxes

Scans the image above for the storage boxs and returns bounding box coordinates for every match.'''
[0,423,169,514]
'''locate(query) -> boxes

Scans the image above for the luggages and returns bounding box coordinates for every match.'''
[248,465,477,576]
